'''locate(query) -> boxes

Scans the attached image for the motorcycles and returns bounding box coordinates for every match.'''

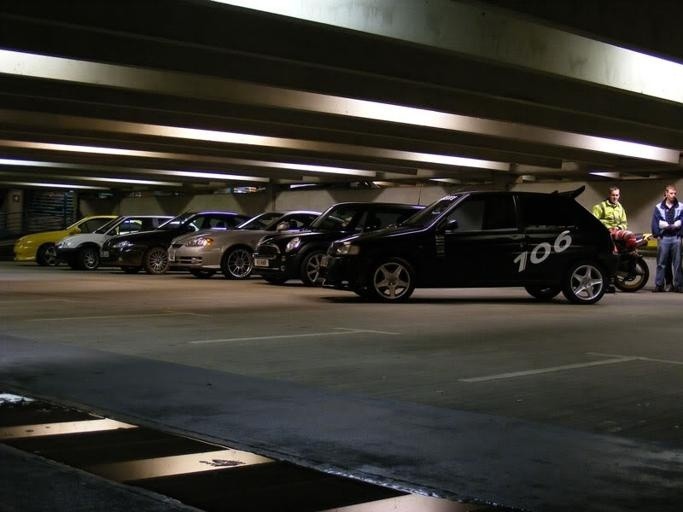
[613,233,648,292]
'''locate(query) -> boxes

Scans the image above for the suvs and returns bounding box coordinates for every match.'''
[330,186,617,303]
[253,201,430,287]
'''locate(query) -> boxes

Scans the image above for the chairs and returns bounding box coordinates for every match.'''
[288,221,296,229]
[202,221,225,229]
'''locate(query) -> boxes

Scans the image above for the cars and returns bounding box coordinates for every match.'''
[99,210,268,275]
[53,213,201,271]
[13,214,144,267]
[166,210,345,280]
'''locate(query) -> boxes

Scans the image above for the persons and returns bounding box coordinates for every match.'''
[591,183,630,294]
[650,183,682,293]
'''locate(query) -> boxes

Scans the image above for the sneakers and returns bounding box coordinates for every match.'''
[607,276,616,293]
[651,284,682,292]
[624,251,643,260]
